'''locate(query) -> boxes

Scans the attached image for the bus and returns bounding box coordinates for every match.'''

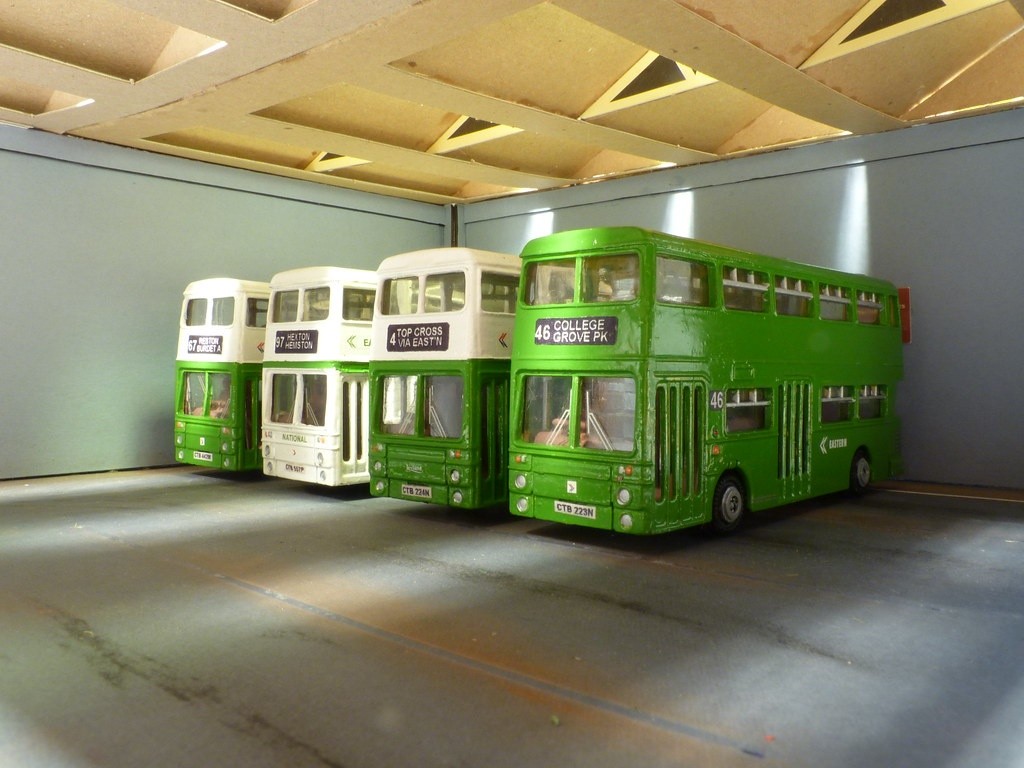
[174,277,274,480]
[259,266,377,498]
[369,247,524,512]
[508,226,905,538]
